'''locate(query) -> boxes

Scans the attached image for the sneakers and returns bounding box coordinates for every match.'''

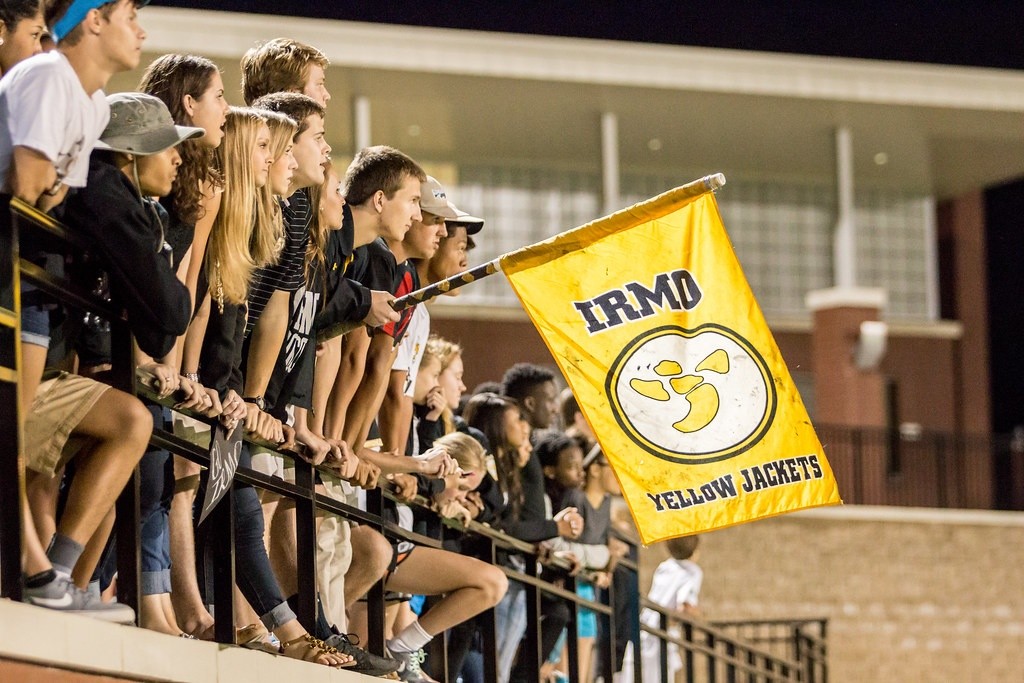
[325,624,402,675]
[385,639,440,683]
[22,576,135,625]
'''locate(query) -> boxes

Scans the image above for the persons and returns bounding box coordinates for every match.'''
[621,534,704,683]
[0,0,641,683]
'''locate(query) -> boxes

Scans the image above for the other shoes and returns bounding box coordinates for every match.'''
[243,632,284,655]
[179,633,199,639]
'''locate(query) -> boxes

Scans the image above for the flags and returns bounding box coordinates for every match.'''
[502,178,843,544]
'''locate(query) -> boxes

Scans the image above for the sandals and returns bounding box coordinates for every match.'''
[205,623,257,645]
[280,633,357,667]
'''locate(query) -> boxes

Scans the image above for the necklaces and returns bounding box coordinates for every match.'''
[214,257,225,314]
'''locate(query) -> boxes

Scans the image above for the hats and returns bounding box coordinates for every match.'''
[464,236,475,250]
[418,174,457,218]
[94,92,205,155]
[443,200,483,235]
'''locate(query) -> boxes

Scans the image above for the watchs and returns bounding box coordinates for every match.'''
[243,396,265,410]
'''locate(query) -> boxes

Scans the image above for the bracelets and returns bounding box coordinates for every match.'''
[184,372,199,382]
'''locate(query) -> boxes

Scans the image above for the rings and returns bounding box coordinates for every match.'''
[165,377,170,382]
[234,400,239,404]
[203,395,209,398]
